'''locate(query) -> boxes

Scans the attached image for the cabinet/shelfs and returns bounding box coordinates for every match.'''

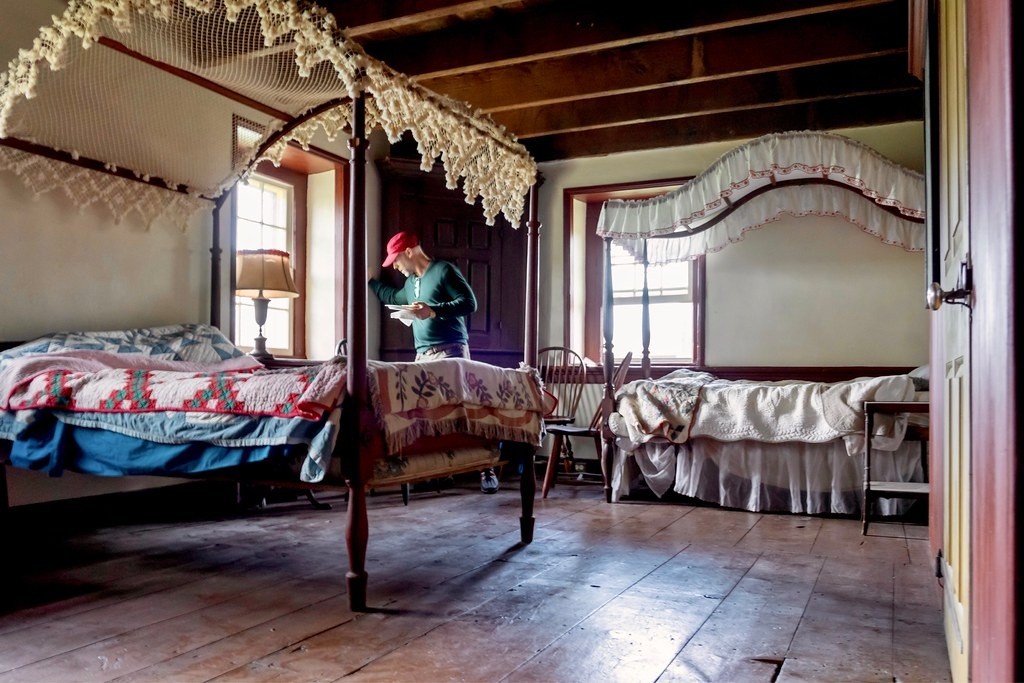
[378,156,529,489]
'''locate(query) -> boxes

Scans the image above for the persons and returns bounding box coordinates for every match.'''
[367,232,499,494]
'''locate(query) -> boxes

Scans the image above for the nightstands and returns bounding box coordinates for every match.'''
[863,400,930,534]
[257,358,328,370]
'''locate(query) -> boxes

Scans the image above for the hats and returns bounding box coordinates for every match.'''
[382,230,420,268]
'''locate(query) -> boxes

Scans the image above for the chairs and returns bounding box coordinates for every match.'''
[335,339,440,506]
[537,347,586,478]
[543,352,632,503]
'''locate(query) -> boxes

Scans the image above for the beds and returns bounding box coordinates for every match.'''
[0,1,545,612]
[596,131,930,514]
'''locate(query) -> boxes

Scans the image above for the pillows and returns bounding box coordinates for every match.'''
[908,362,929,392]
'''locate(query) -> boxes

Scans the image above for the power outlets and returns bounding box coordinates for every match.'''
[574,462,586,472]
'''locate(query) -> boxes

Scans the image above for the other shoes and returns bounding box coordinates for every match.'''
[480,468,499,494]
[414,476,453,491]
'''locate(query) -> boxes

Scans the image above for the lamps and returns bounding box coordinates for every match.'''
[235,249,300,359]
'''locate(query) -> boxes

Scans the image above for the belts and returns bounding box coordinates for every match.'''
[426,342,460,355]
